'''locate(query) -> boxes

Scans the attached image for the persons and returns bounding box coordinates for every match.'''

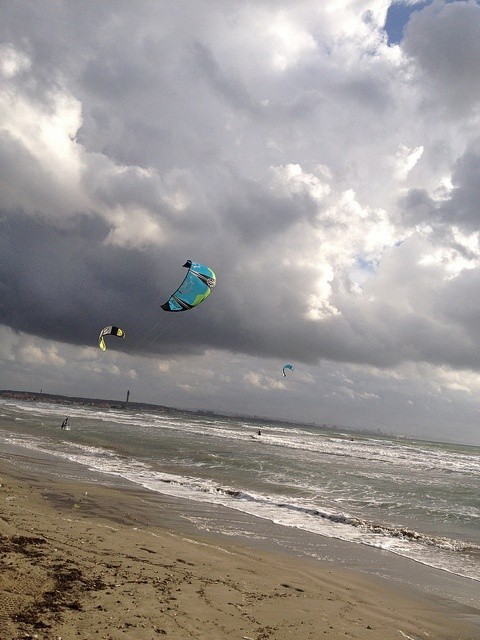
[258,429,261,436]
[61,417,68,429]
